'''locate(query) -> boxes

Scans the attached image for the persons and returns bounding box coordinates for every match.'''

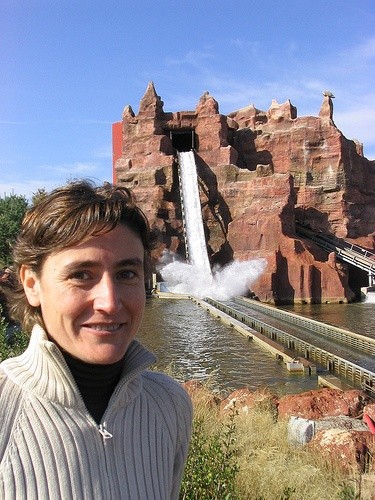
[0,179,195,500]
